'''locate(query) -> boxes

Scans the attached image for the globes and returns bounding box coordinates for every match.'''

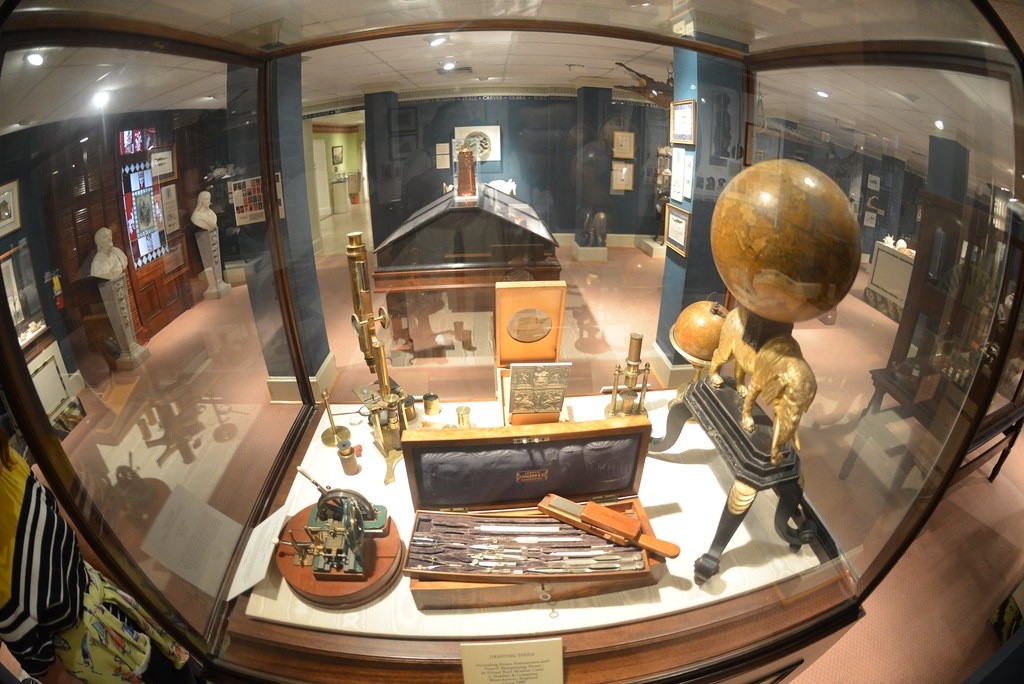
[710,159,863,354]
[667,290,731,424]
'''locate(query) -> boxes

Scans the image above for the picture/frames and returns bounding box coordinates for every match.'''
[146,143,179,185]
[0,177,23,240]
[610,161,635,191]
[663,202,692,260]
[612,130,635,160]
[669,98,697,146]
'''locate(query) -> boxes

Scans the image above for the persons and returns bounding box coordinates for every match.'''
[90,227,128,280]
[0,399,197,683]
[191,191,217,229]
